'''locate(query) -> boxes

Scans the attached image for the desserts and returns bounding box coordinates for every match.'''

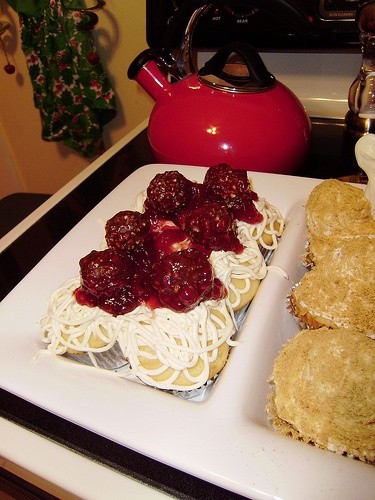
[39,162,285,391]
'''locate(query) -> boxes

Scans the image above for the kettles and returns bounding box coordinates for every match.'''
[127,40,311,175]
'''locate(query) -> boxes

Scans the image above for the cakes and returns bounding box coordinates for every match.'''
[267,178,375,463]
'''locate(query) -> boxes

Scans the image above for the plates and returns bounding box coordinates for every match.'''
[0,165,373,500]
